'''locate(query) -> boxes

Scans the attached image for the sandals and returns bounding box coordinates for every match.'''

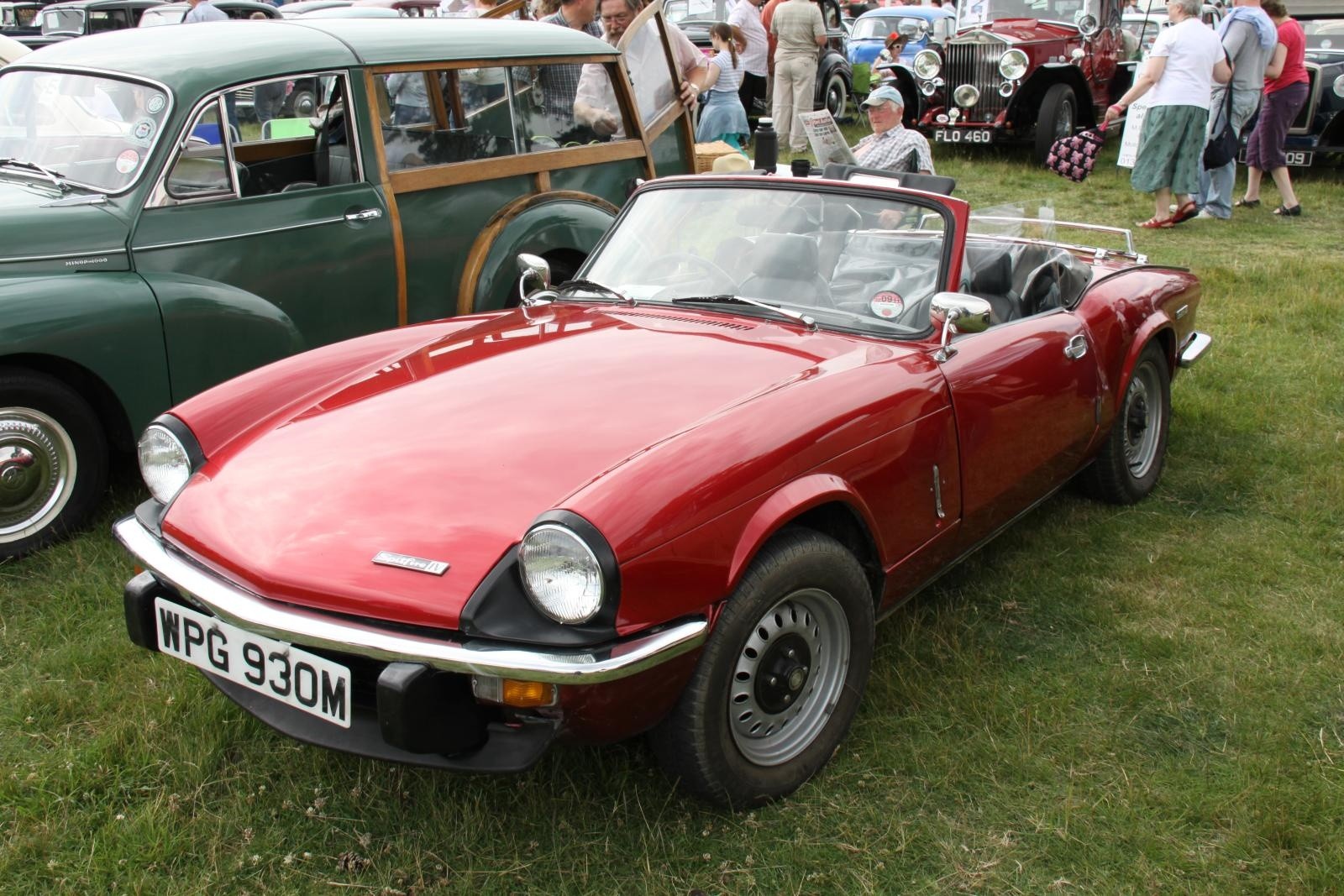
[1170,201,1201,224]
[1134,217,1180,230]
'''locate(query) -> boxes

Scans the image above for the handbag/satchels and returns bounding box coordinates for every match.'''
[1203,123,1241,171]
[1043,105,1122,183]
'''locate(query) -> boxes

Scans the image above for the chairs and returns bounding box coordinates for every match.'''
[738,234,835,309]
[902,245,1026,334]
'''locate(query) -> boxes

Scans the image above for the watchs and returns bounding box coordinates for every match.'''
[690,83,699,92]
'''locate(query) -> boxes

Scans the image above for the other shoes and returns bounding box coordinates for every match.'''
[1169,205,1178,214]
[1272,204,1301,218]
[1194,208,1217,220]
[1234,196,1265,208]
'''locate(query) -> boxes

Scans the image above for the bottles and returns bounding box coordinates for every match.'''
[754,117,778,173]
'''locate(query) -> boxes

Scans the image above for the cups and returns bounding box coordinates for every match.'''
[791,159,810,177]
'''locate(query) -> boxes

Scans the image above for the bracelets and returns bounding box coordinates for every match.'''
[1118,99,1126,107]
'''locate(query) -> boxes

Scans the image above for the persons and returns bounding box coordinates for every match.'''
[179,0,242,142]
[727,0,827,153]
[1105,0,1311,228]
[868,32,910,78]
[825,0,959,29]
[384,72,432,124]
[849,86,936,230]
[250,11,287,139]
[457,0,512,150]
[512,0,712,146]
[695,22,751,160]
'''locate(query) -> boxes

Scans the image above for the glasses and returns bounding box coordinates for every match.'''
[599,15,629,27]
[893,44,904,48]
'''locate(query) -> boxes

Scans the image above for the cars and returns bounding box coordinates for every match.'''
[659,1,1344,172]
[1,0,692,557]
[110,173,1215,816]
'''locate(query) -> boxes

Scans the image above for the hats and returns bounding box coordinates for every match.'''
[884,33,910,51]
[859,85,905,108]
